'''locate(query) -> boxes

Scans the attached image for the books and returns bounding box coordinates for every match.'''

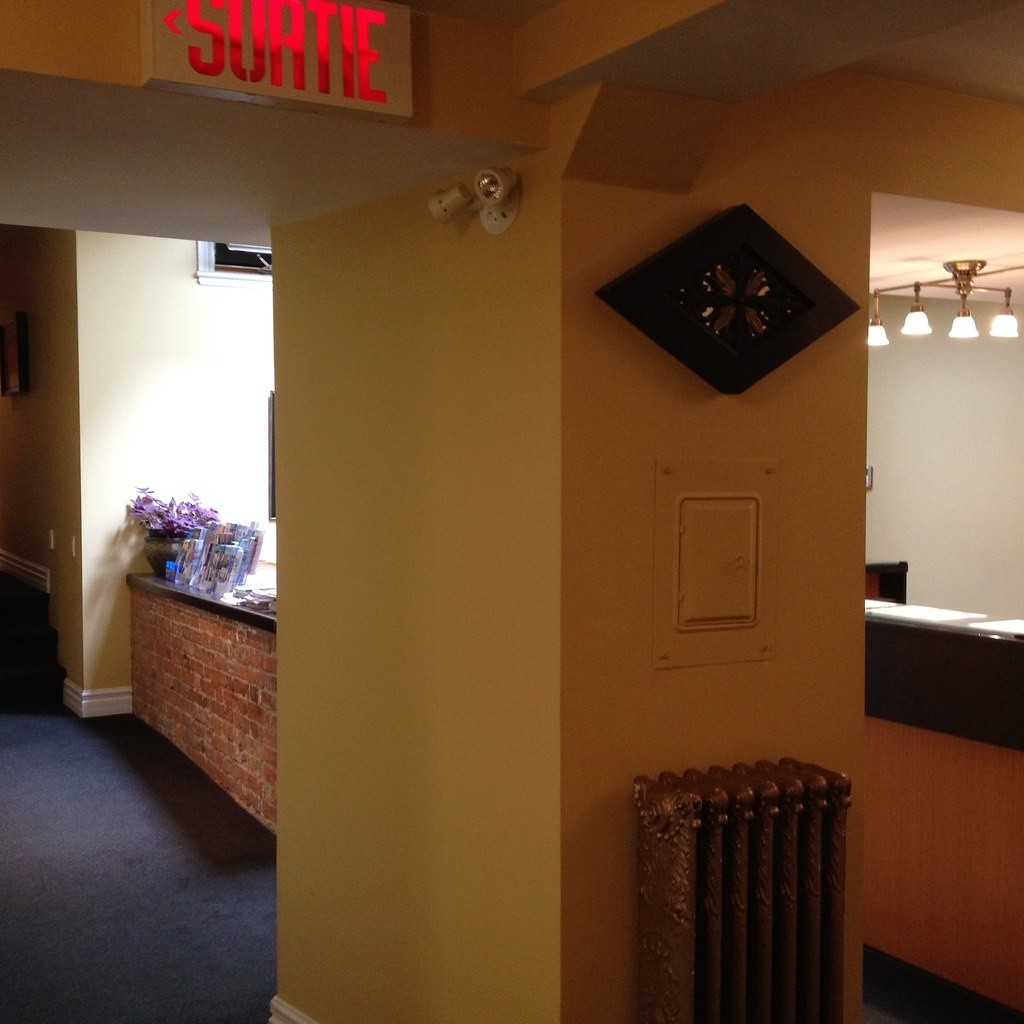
[221,584,278,615]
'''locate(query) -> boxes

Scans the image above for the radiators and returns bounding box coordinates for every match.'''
[630,757,853,1024]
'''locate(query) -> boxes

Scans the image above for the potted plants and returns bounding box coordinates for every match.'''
[128,486,221,577]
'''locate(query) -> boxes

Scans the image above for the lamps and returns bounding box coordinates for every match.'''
[426,166,522,235]
[867,260,1023,347]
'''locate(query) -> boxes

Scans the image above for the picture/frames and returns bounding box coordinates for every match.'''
[596,203,862,394]
[0,311,30,397]
